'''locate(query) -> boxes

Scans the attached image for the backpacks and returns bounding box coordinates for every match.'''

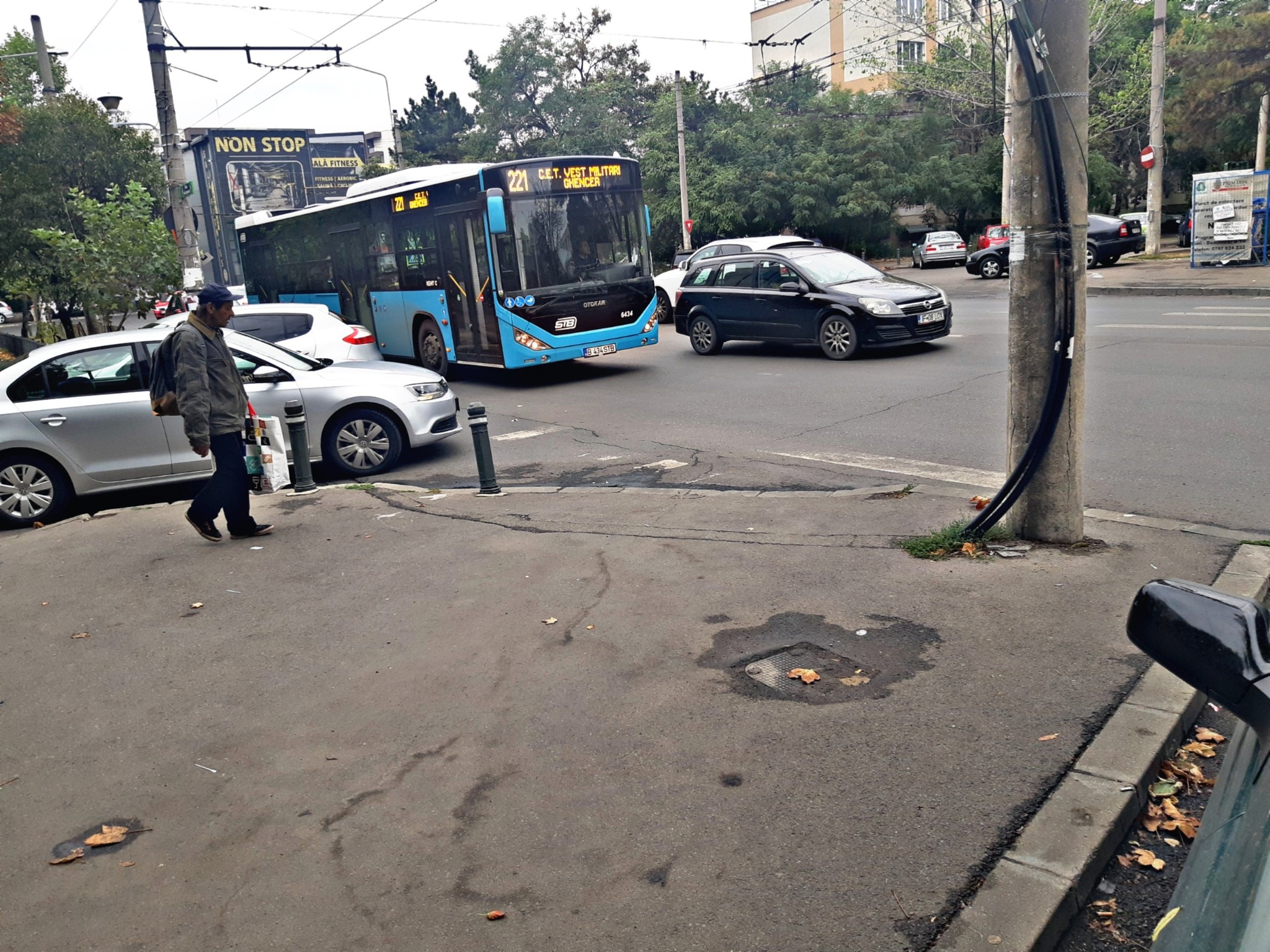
[149,320,185,416]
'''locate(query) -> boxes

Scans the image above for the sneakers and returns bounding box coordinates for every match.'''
[184,507,222,541]
[228,516,274,540]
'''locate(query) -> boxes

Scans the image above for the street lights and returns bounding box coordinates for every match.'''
[334,60,405,170]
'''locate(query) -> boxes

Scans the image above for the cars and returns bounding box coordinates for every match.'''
[654,235,952,360]
[977,224,1011,251]
[911,230,968,269]
[0,302,463,528]
[0,301,14,324]
[28,295,84,322]
[1161,204,1192,248]
[153,290,200,320]
[965,211,1146,279]
[1122,576,1270,952]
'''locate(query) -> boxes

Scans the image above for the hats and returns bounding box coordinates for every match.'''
[197,282,244,304]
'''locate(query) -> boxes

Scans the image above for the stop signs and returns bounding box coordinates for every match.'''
[1139,145,1155,168]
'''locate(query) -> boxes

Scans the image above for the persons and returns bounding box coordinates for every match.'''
[172,282,275,540]
[567,240,602,280]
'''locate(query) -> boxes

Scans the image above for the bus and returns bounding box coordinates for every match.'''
[234,154,659,380]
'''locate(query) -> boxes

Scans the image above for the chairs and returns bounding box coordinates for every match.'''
[762,270,781,288]
[724,272,746,286]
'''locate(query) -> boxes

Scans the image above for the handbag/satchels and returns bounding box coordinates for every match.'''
[241,400,291,495]
